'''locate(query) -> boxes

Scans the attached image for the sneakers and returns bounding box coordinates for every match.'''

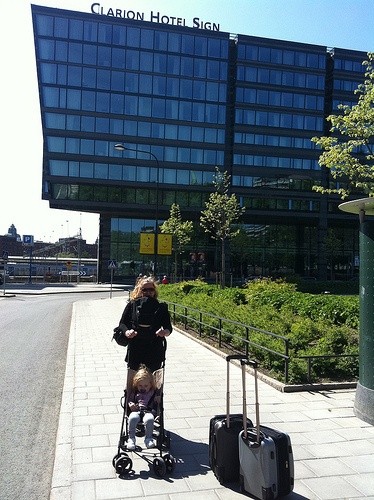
[144,439,155,448]
[126,439,136,450]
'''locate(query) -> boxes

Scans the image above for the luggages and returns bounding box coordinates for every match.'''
[210,352,255,485]
[240,357,295,499]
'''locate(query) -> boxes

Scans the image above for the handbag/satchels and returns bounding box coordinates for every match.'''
[112,300,137,347]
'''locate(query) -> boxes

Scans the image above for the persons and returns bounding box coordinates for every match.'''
[112,276,173,438]
[126,367,157,452]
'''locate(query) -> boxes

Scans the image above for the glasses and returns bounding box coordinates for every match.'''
[141,288,155,292]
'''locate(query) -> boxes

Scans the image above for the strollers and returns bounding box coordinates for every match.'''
[111,330,176,480]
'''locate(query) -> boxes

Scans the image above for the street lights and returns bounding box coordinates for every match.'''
[113,143,161,274]
